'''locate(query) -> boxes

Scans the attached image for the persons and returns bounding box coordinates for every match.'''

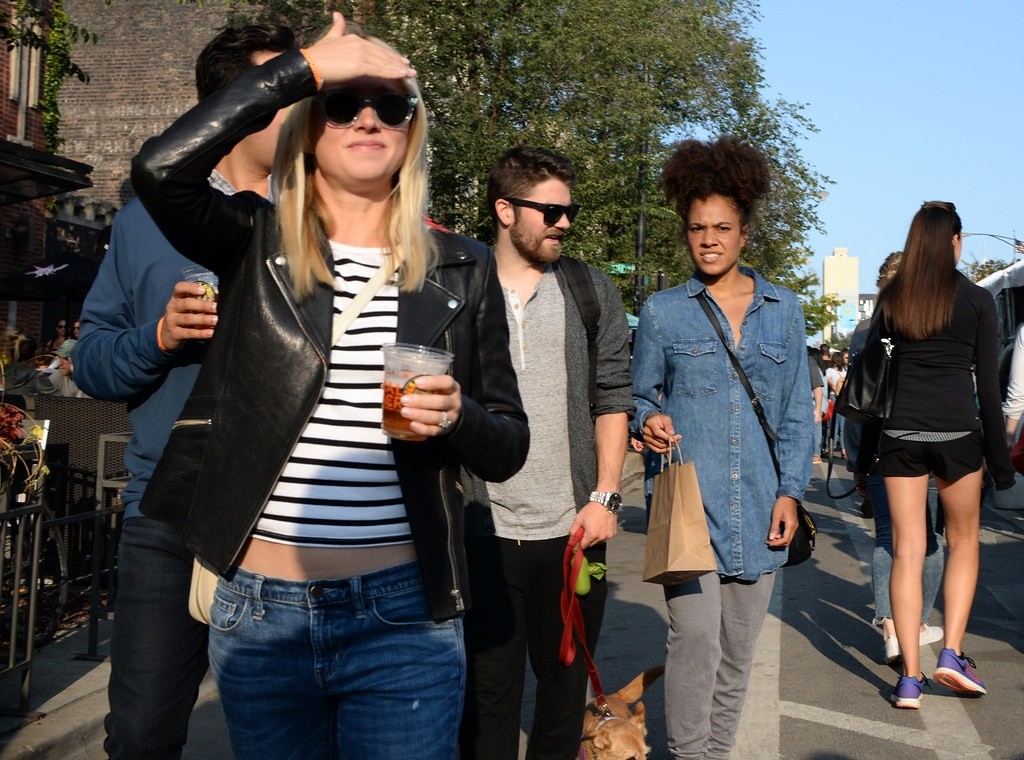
[629,135,813,760]
[72,25,303,760]
[130,10,530,760]
[630,252,1024,665]
[458,146,634,760]
[4,319,92,399]
[867,200,1016,708]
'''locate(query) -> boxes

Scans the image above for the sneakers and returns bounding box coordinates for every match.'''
[890,671,933,709]
[919,623,944,645]
[935,645,989,696]
[885,638,902,665]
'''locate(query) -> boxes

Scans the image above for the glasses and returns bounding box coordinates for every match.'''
[73,327,79,331]
[313,86,418,131]
[502,197,581,225]
[56,325,66,329]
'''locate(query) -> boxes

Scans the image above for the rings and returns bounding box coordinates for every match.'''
[438,410,451,429]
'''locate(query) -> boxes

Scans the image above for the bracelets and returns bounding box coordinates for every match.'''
[300,48,321,90]
[157,317,166,351]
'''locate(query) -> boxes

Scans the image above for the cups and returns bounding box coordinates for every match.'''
[181,263,219,343]
[379,342,455,441]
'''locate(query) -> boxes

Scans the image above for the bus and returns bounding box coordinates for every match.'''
[849,258,1024,360]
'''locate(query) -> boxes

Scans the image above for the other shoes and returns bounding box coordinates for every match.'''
[811,455,822,464]
[842,451,846,459]
[860,500,873,518]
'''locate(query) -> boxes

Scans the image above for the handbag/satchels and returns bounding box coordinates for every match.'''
[822,399,834,422]
[836,285,901,424]
[188,550,219,624]
[642,431,718,586]
[775,501,818,568]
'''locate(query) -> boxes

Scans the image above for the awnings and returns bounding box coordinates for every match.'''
[0,138,94,208]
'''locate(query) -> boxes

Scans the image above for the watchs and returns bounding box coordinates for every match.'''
[590,491,623,516]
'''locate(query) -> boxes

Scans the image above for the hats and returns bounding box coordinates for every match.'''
[52,339,78,361]
